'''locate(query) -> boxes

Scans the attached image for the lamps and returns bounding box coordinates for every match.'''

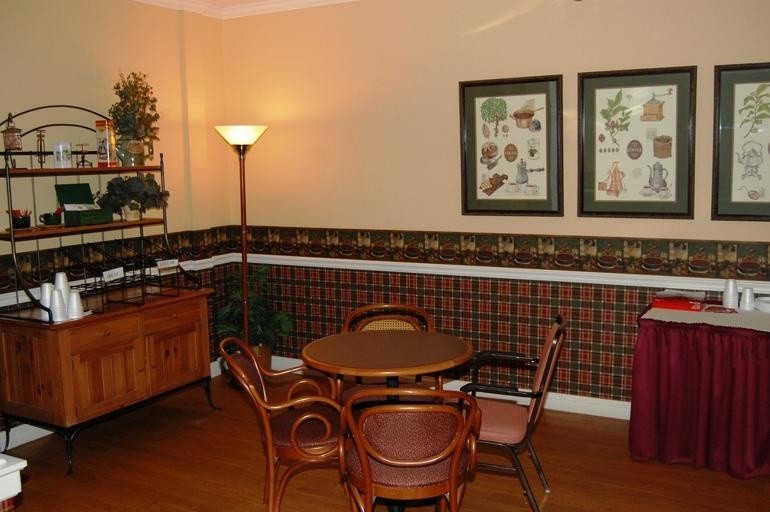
[214,121,270,353]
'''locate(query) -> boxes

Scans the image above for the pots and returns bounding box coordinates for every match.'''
[513,106,544,129]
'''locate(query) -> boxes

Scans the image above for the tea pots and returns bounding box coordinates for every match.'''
[646,160,668,193]
[114,136,155,166]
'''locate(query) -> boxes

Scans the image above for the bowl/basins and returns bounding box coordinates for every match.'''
[0,239,239,289]
[251,226,763,277]
[8,216,31,228]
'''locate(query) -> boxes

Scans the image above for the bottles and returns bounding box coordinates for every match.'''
[515,158,528,184]
[487,156,502,170]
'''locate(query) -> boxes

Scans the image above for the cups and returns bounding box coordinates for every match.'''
[659,187,669,195]
[756,296,770,308]
[526,184,539,195]
[507,182,518,193]
[722,279,755,311]
[653,136,672,158]
[38,270,84,323]
[38,213,62,225]
[526,138,541,160]
[641,185,652,194]
[51,142,72,168]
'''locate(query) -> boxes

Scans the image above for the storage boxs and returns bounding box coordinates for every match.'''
[53,179,115,228]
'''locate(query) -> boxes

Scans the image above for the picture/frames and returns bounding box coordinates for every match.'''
[573,64,698,222]
[454,72,566,219]
[709,62,768,224]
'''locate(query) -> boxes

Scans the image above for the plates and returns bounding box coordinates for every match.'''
[6,226,37,232]
[754,305,770,312]
[34,224,65,230]
[657,193,672,199]
[639,191,653,196]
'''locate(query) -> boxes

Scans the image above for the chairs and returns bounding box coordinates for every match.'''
[213,334,366,511]
[336,378,485,512]
[440,307,572,512]
[337,299,449,408]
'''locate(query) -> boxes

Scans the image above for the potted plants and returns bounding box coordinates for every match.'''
[208,261,298,390]
[102,69,158,155]
[90,172,173,226]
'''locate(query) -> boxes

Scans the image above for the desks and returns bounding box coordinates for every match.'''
[627,298,770,485]
[299,326,480,510]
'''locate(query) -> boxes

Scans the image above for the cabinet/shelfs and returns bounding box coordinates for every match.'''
[0,105,206,324]
[0,277,218,476]
